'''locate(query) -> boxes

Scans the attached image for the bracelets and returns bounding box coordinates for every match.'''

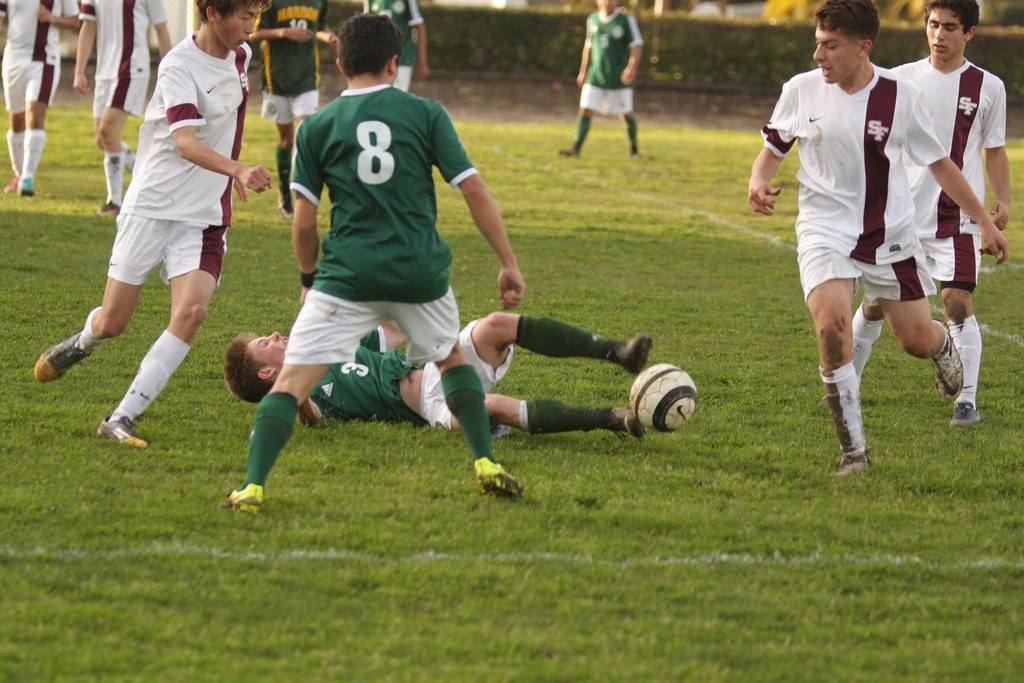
[301,267,319,288]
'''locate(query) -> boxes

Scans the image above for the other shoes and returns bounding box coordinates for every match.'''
[16,177,35,197]
[277,186,292,219]
[2,176,20,193]
[97,202,122,216]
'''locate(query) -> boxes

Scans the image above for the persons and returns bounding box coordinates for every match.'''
[72,0,173,218]
[224,13,525,516]
[32,0,273,449]
[850,0,1011,427]
[746,0,1009,478]
[0,0,80,197]
[558,0,644,158]
[221,308,655,444]
[362,0,430,93]
[246,0,342,220]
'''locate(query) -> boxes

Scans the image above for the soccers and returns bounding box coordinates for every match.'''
[630,363,698,433]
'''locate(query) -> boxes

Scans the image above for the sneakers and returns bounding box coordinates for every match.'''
[828,453,871,477]
[597,406,644,439]
[927,318,965,400]
[93,415,149,450]
[473,456,524,498]
[949,401,981,427]
[218,482,264,517]
[602,335,652,375]
[33,332,91,383]
